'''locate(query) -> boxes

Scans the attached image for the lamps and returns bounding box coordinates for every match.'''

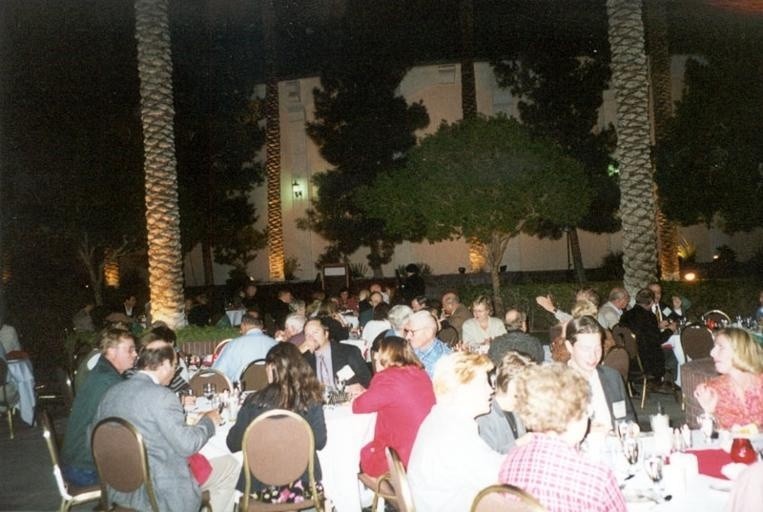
[292,179,301,198]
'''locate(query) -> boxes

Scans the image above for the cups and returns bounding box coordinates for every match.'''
[651,414,669,442]
[622,439,639,466]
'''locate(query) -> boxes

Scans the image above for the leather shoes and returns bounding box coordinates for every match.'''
[660,381,681,390]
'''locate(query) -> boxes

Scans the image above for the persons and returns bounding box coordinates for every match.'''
[1,264,762,512]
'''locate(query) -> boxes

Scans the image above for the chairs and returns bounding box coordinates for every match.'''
[539,311,763,413]
[186,368,235,393]
[33,334,93,415]
[233,409,324,512]
[383,446,419,511]
[240,359,269,393]
[467,486,548,510]
[41,414,99,510]
[89,418,161,512]
[1,339,35,441]
[211,337,231,365]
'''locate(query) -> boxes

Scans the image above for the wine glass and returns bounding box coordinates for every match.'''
[322,386,334,413]
[700,415,713,448]
[643,454,665,497]
[204,384,216,408]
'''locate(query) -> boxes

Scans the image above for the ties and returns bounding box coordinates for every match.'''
[654,306,660,323]
[319,356,331,385]
